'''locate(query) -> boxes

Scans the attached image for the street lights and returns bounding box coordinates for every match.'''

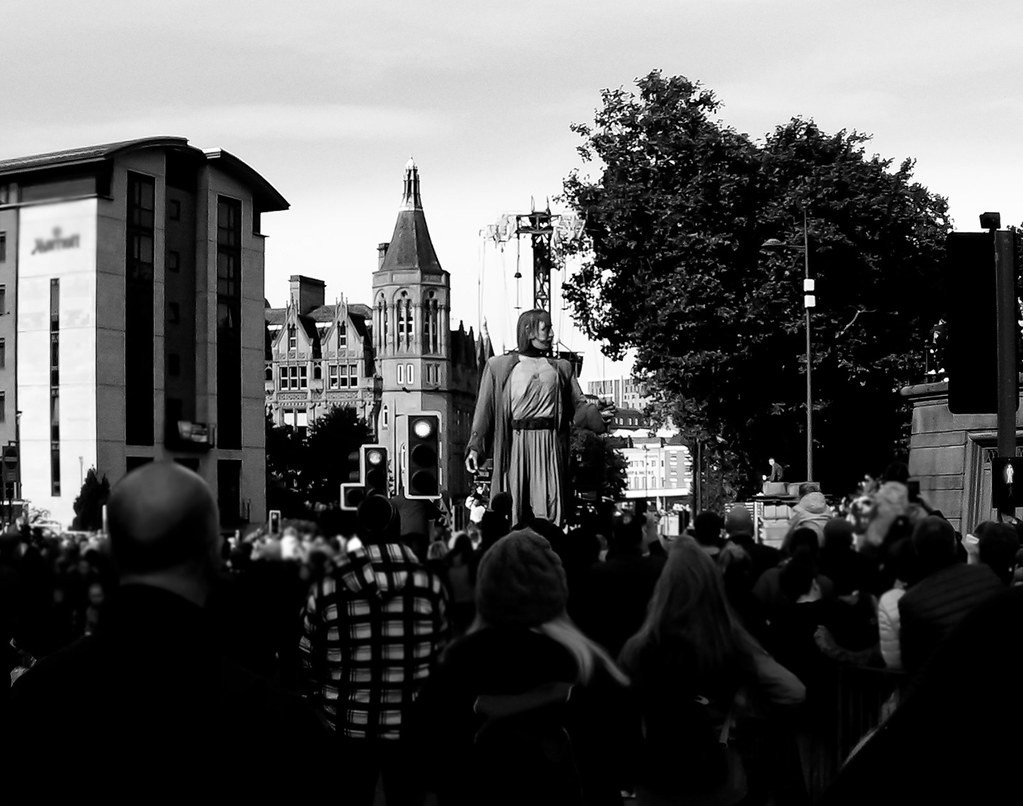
[761,211,815,481]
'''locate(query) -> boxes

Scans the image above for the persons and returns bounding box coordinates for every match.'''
[0,462,1022,806]
[756,458,784,496]
[464,310,617,530]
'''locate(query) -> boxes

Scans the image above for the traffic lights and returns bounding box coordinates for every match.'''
[405,410,443,500]
[340,444,390,512]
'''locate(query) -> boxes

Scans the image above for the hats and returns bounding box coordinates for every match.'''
[789,492,833,525]
[475,527,568,634]
[353,494,401,545]
[905,515,968,569]
